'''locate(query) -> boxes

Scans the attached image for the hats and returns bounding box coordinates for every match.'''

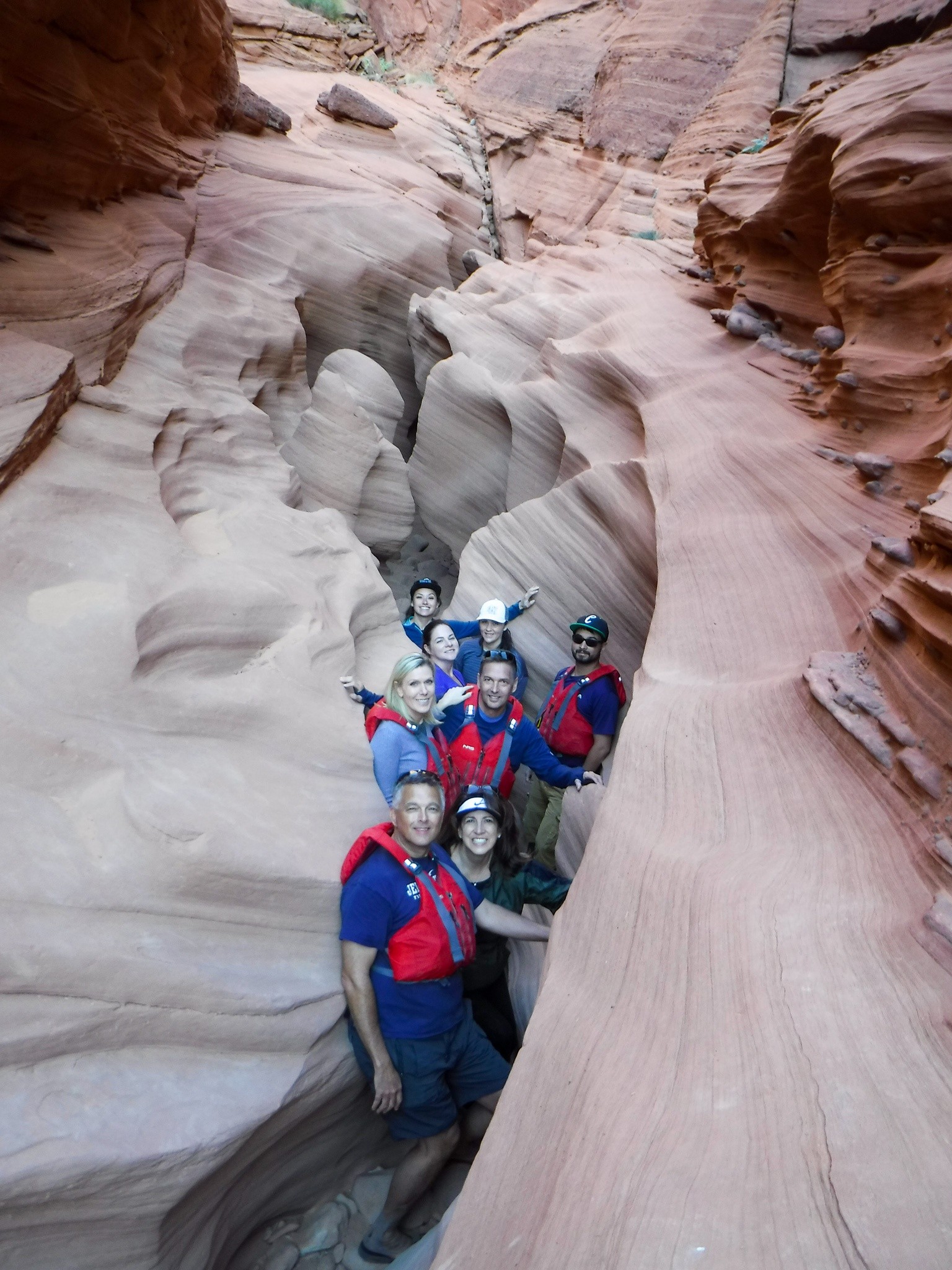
[455,797,504,821]
[569,614,610,643]
[476,598,509,624]
[411,577,442,601]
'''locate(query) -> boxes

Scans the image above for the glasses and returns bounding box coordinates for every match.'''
[463,783,499,796]
[483,650,518,671]
[572,634,603,647]
[397,769,443,786]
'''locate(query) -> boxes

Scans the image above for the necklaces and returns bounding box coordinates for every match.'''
[455,853,492,886]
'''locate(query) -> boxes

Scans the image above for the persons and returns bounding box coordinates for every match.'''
[338,648,605,809]
[522,613,626,871]
[340,619,467,744]
[338,770,573,1264]
[452,597,529,701]
[402,577,540,650]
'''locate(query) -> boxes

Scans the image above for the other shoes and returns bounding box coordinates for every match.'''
[358,1230,447,1266]
[447,1138,480,1165]
